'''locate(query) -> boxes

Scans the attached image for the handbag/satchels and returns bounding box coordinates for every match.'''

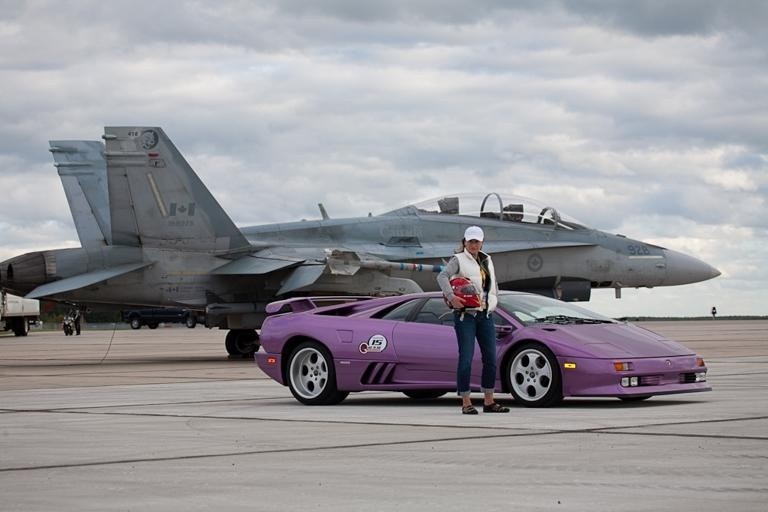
[442,277,480,311]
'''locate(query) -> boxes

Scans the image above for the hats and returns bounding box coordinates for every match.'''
[464,226,484,243]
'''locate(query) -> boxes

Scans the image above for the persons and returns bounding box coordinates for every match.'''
[435,225,510,415]
[69,307,81,336]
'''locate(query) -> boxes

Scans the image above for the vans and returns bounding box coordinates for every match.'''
[121,305,207,329]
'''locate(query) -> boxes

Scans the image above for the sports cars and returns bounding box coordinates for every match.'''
[253,286,715,408]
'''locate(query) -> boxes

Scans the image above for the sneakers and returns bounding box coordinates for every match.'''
[462,405,478,414]
[483,403,509,413]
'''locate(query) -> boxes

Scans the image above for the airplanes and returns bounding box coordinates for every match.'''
[1,125,723,360]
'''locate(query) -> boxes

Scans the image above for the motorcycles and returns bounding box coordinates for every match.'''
[61,316,75,337]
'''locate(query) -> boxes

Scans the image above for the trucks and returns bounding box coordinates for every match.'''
[0,289,41,336]
